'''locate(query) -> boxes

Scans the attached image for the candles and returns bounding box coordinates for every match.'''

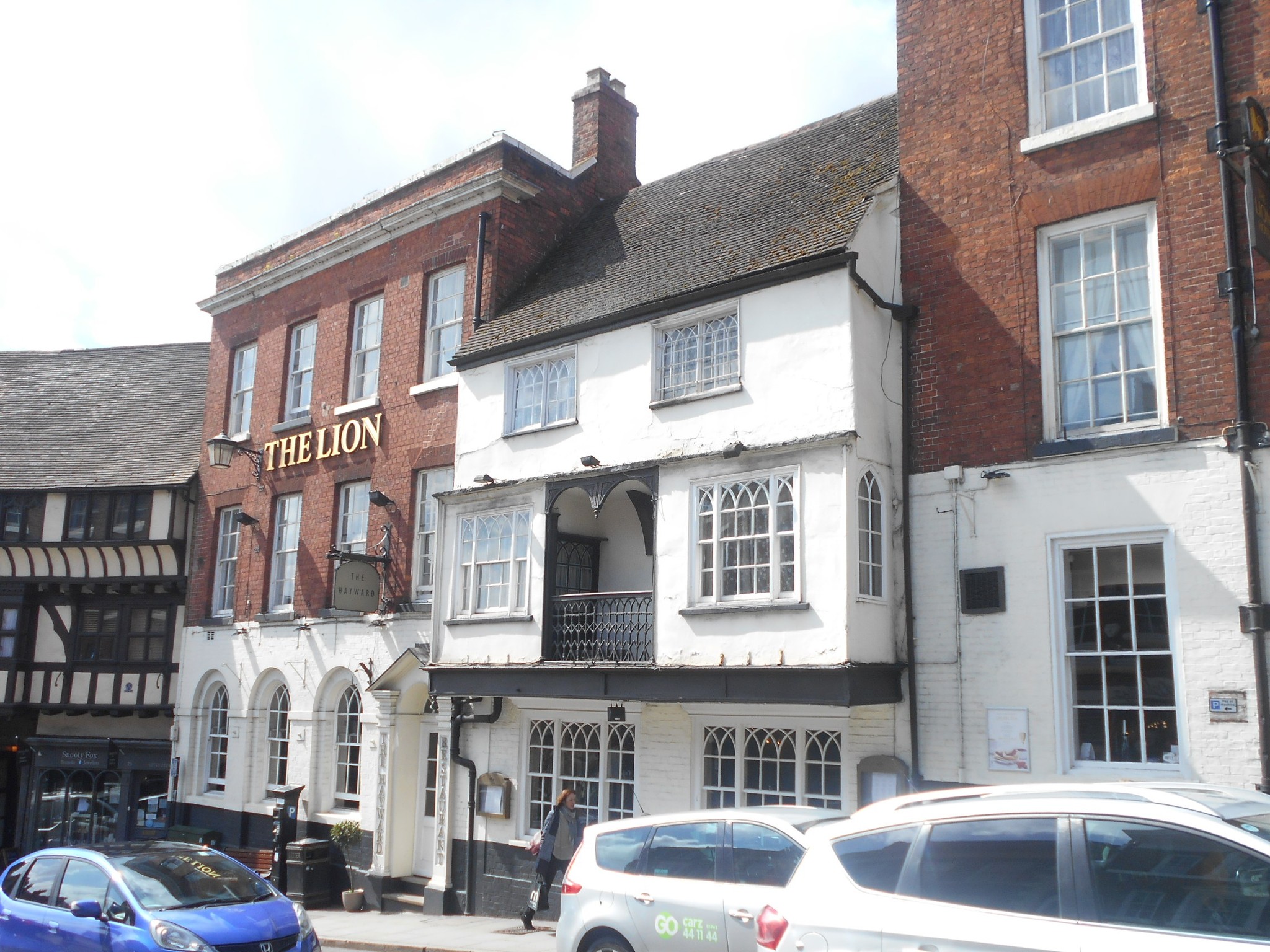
[1124,720,1126,736]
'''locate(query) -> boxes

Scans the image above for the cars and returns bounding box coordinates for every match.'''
[39,789,144,844]
[0,840,323,952]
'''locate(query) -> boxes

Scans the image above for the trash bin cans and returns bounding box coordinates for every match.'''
[285,838,335,911]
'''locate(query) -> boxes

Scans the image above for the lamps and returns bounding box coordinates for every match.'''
[723,441,743,459]
[368,490,398,512]
[293,624,310,631]
[607,700,626,722]
[233,629,247,635]
[581,455,602,468]
[474,475,494,485]
[235,512,259,526]
[206,428,263,481]
[367,620,385,627]
[980,470,1011,480]
[12,736,20,753]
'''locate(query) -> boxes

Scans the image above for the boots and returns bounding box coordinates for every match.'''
[520,907,535,931]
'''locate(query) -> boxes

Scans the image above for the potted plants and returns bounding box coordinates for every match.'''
[330,821,365,911]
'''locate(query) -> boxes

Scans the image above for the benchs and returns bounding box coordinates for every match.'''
[222,846,274,880]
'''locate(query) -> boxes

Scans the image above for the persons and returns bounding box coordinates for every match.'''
[521,789,578,930]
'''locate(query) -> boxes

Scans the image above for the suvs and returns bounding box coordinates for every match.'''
[555,805,854,952]
[754,779,1270,952]
